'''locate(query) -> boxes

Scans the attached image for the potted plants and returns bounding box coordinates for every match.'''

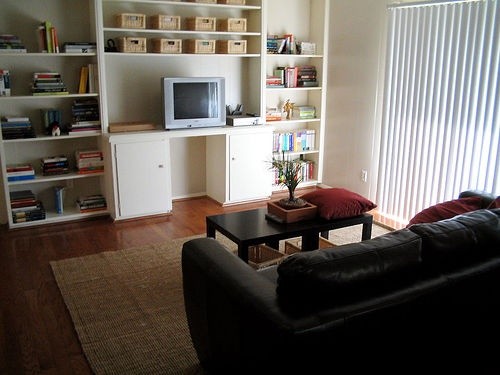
[265,158,305,207]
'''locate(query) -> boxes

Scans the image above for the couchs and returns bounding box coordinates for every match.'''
[180,192,500,375]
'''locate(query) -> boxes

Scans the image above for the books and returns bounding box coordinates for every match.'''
[292,105,316,120]
[10,189,46,224]
[76,194,108,214]
[7,162,35,182]
[266,107,282,121]
[74,148,105,174]
[40,154,70,176]
[273,129,316,153]
[267,64,319,87]
[1,97,102,141]
[272,158,314,184]
[267,34,318,55]
[1,62,99,97]
[0,20,97,52]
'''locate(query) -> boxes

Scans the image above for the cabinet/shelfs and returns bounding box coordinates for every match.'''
[264,0,331,191]
[97,140,171,219]
[95,0,265,134]
[0,0,110,229]
[205,132,273,202]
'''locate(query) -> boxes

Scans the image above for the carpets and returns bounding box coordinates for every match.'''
[49,223,397,374]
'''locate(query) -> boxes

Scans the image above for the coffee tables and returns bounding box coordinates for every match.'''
[204,207,373,264]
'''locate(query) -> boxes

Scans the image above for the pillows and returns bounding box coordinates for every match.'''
[298,188,378,221]
[405,197,483,228]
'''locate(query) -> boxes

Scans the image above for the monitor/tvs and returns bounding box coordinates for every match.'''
[161,76,226,129]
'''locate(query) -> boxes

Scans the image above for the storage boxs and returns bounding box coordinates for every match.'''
[114,0,246,55]
[233,245,287,269]
[285,235,338,255]
[227,114,257,127]
